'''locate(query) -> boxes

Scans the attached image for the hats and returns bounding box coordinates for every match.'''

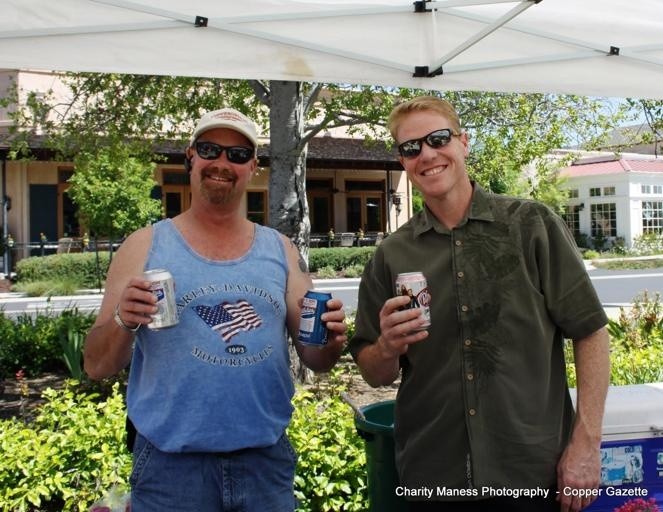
[189,108,258,160]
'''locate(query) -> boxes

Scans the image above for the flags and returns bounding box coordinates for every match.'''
[191,299,262,344]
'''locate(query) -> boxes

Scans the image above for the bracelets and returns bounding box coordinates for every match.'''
[112,302,142,333]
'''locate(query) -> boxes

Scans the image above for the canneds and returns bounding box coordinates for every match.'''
[143,270,179,332]
[295,289,332,348]
[395,271,433,331]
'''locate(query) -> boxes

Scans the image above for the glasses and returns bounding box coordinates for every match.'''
[398,128,462,158]
[193,141,254,164]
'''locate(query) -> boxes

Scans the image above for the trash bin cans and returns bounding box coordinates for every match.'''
[354,400,405,511]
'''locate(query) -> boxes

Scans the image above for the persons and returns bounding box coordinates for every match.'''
[400,284,408,295]
[83,107,349,512]
[345,95,611,512]
[405,287,420,310]
